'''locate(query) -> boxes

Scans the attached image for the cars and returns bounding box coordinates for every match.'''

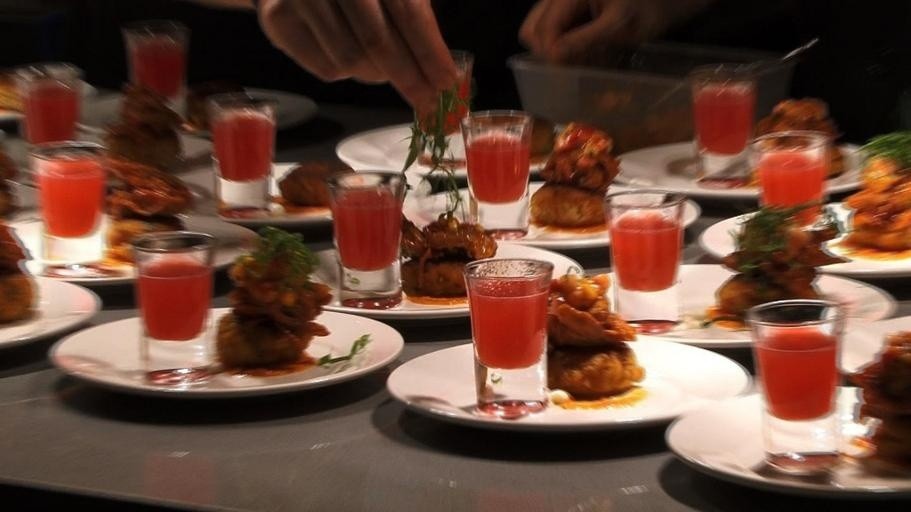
[743,292,848,476]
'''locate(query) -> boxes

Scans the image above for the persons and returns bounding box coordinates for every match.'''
[258,2,714,121]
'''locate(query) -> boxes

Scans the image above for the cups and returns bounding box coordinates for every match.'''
[332,171,410,310]
[20,16,279,210]
[602,190,688,334]
[28,141,105,265]
[462,258,554,416]
[689,61,757,186]
[743,292,848,476]
[463,109,532,243]
[751,131,827,232]
[134,232,219,372]
[413,50,472,176]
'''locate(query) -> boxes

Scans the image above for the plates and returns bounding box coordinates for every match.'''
[1,79,317,204]
[835,314,910,383]
[335,122,568,178]
[171,163,337,223]
[6,213,264,283]
[2,275,100,345]
[614,141,871,200]
[301,242,583,318]
[403,180,699,249]
[49,307,403,397]
[384,332,751,428]
[661,383,910,494]
[590,263,895,349]
[696,201,910,278]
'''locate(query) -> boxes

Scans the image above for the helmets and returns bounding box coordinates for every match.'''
[661,383,910,494]
